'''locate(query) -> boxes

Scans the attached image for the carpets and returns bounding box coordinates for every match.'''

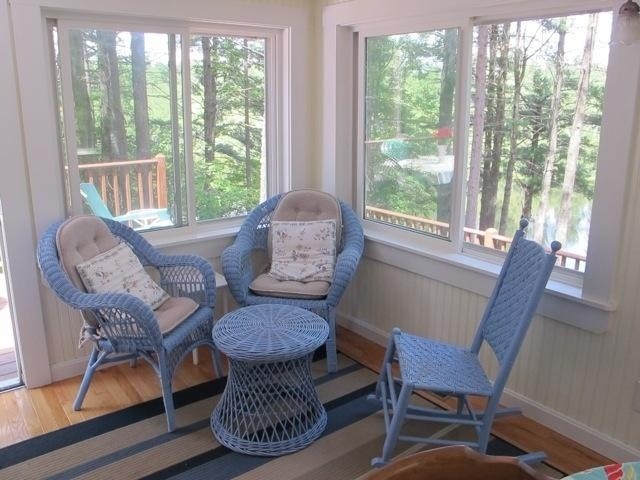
[1,343,566,479]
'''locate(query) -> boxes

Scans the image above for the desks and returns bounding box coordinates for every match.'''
[210,302,330,455]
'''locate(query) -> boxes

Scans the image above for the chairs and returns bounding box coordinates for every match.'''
[219,190,365,373]
[39,215,216,433]
[80,181,169,229]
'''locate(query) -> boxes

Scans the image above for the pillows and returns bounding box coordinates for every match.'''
[78,242,170,323]
[270,220,338,284]
